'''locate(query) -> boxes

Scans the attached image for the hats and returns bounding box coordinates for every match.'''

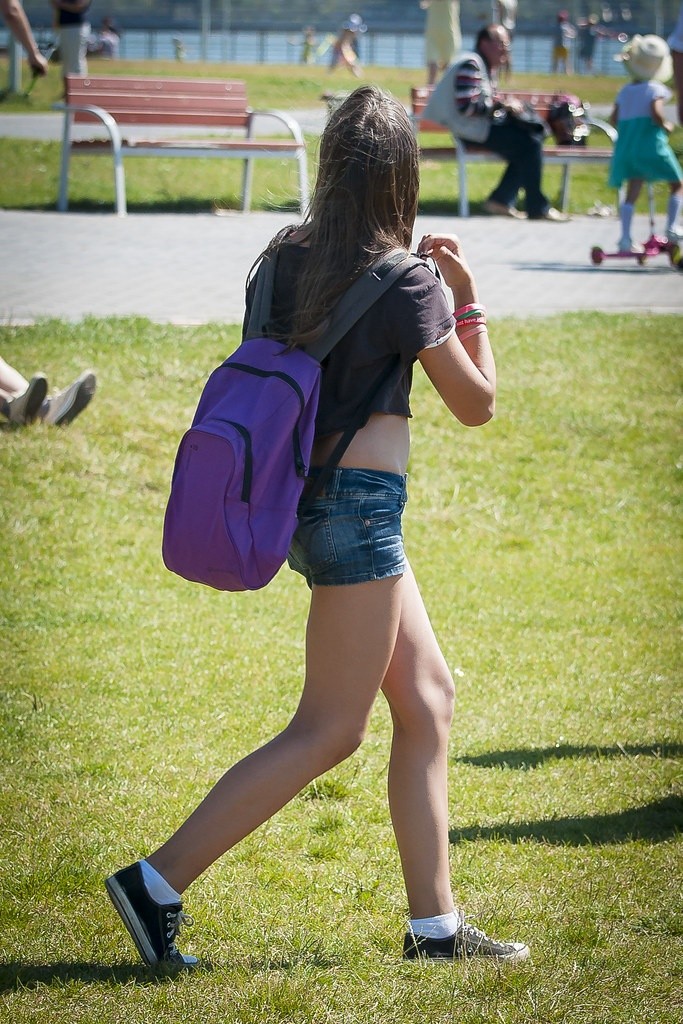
[622,34,673,84]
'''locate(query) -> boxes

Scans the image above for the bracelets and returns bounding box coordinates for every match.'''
[452,303,488,341]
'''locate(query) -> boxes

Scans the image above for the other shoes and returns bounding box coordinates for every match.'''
[664,225,683,240]
[619,237,646,254]
[45,372,99,424]
[489,198,526,222]
[546,208,570,219]
[6,371,49,426]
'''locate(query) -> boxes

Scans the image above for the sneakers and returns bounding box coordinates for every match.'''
[105,861,199,974]
[404,917,529,964]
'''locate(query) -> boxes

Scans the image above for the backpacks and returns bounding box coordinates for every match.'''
[162,227,429,591]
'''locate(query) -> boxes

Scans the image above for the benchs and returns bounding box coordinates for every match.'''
[414,88,618,219]
[53,75,311,219]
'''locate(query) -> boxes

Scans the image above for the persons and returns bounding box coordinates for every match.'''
[104,84,531,969]
[302,26,314,64]
[420,0,461,85]
[172,37,183,62]
[423,24,571,221]
[606,33,683,253]
[666,3,683,124]
[329,25,359,68]
[550,10,574,74]
[0,354,97,427]
[490,0,518,80]
[577,13,612,72]
[88,16,120,58]
[50,0,92,76]
[0,0,48,78]
